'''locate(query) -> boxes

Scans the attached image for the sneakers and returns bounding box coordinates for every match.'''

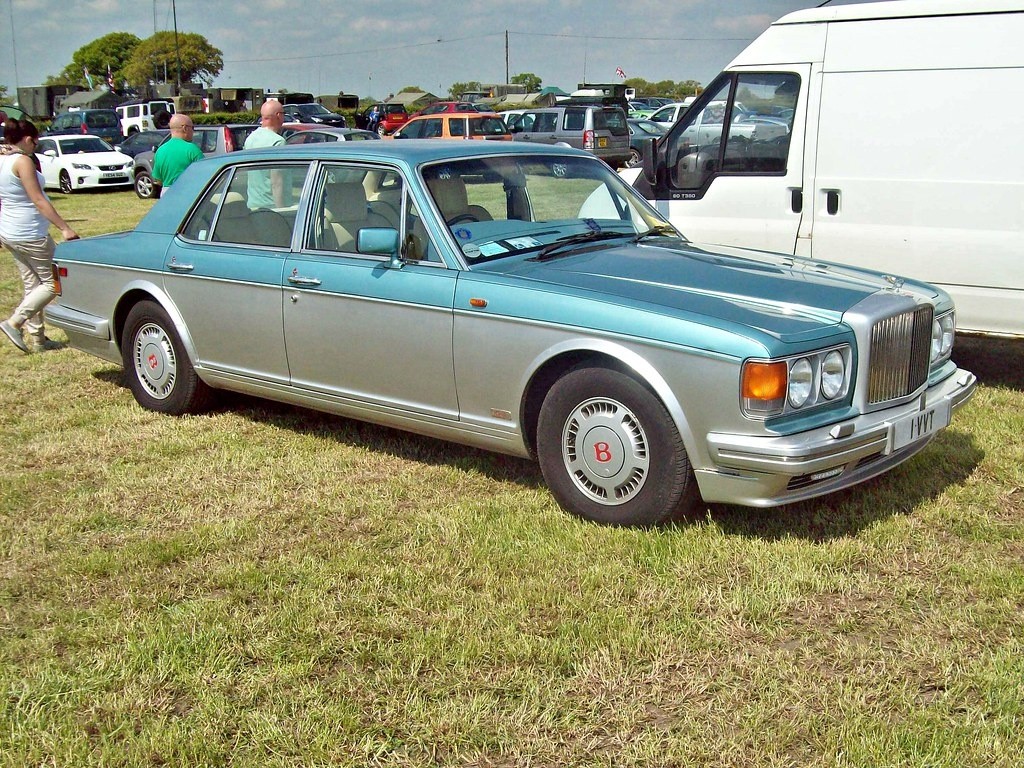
[33,336,62,351]
[0,319,29,352]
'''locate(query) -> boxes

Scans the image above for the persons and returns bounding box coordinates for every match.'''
[153,114,205,199]
[244,100,291,211]
[367,106,380,132]
[0,118,80,352]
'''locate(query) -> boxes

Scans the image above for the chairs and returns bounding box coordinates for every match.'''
[191,192,292,248]
[324,185,396,252]
[414,178,493,263]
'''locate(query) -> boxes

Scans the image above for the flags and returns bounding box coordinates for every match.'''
[107,64,116,94]
[615,67,626,78]
[83,66,94,90]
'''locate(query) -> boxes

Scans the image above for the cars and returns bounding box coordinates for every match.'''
[46,134,978,522]
[33,134,129,194]
[0,82,781,192]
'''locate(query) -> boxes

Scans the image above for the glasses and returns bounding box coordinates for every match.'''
[182,125,194,130]
[277,112,286,115]
[32,139,38,149]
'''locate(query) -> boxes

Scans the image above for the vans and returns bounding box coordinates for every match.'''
[576,0,1024,342]
